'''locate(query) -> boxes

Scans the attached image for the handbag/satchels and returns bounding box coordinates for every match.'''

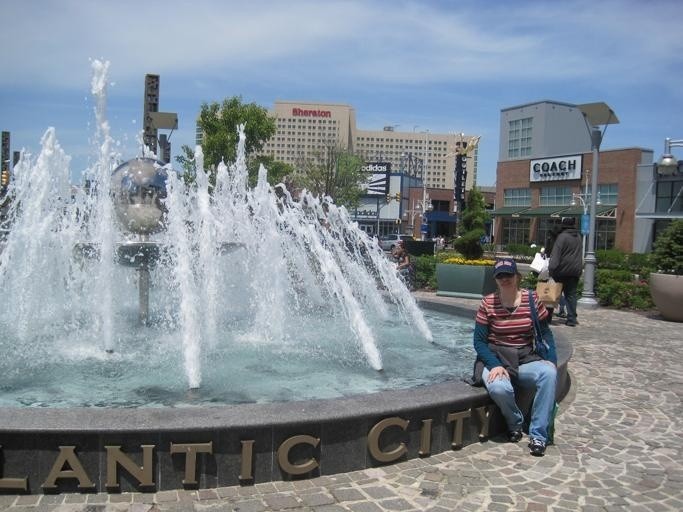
[535,278,563,303]
[529,251,550,274]
[527,289,557,366]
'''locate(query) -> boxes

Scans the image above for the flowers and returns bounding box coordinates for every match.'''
[443,257,496,266]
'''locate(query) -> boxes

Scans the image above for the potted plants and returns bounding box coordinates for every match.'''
[645,217,683,322]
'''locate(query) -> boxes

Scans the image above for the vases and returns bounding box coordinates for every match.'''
[436,263,499,299]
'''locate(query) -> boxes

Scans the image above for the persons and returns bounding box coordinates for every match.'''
[533,217,583,326]
[395,233,494,290]
[473,258,557,454]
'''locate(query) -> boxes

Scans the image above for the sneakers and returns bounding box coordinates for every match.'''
[559,311,579,326]
[507,428,523,442]
[528,437,545,456]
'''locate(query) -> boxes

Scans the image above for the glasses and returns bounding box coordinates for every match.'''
[495,272,514,279]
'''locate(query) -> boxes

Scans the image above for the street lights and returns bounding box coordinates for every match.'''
[657,138,683,175]
[402,198,434,241]
[570,191,602,265]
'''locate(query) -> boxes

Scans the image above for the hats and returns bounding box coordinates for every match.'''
[493,258,517,277]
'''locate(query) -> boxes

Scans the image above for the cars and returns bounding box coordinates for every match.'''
[380,234,414,251]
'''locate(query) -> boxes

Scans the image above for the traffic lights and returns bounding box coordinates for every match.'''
[396,192,401,202]
[386,194,390,203]
[2,171,9,186]
